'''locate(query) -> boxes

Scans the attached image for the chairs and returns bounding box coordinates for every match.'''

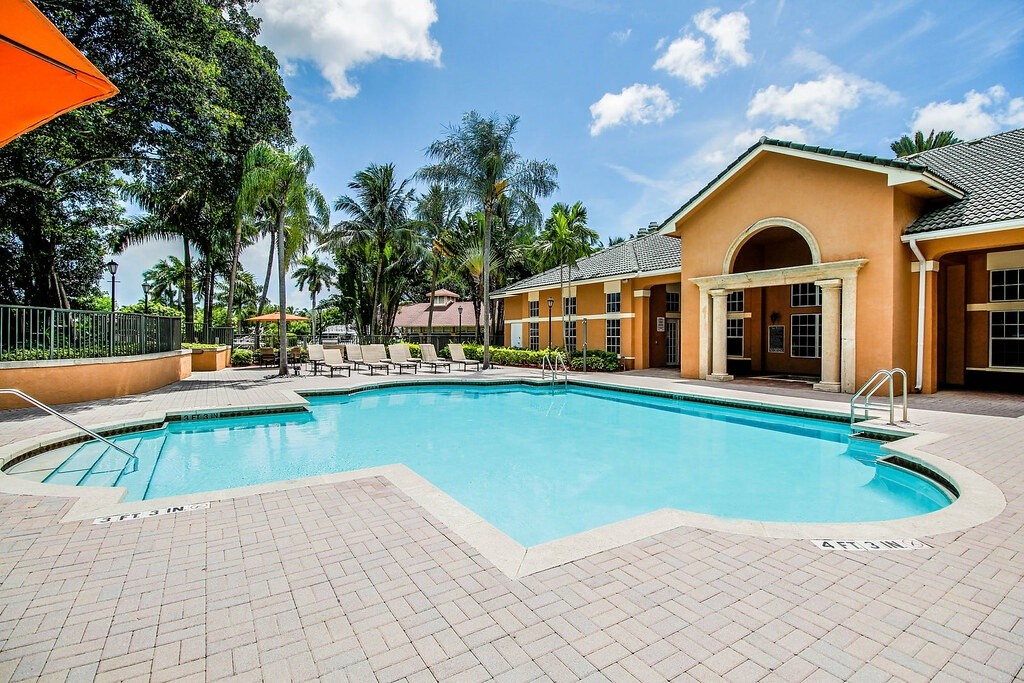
[259,344,480,380]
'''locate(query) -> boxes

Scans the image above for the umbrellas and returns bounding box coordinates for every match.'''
[244,312,307,342]
[0,0,120,148]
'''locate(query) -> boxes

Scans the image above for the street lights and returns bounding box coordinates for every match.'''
[458,305,464,344]
[107,259,119,324]
[317,306,322,345]
[547,296,554,351]
[142,280,151,314]
